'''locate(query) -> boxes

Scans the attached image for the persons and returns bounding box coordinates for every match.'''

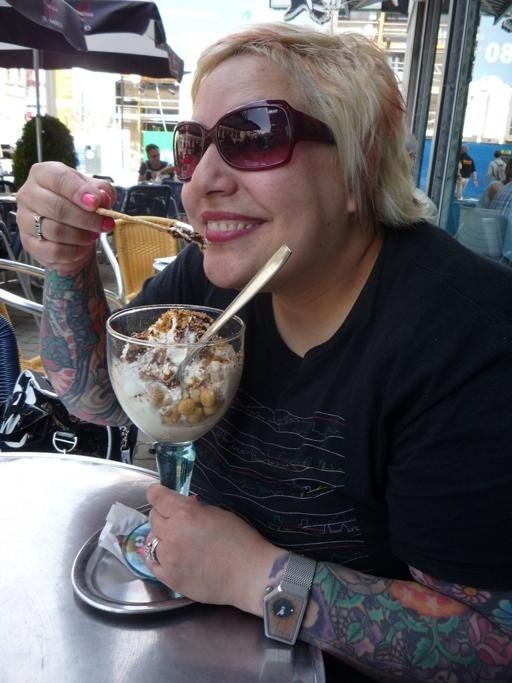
[456,143,478,201]
[488,150,507,182]
[15,25,512,683]
[476,159,512,209]
[137,143,175,183]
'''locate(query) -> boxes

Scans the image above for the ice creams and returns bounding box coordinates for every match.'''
[112,308,242,444]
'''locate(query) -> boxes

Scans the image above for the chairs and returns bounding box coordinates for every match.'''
[455,201,506,261]
[0,162,202,463]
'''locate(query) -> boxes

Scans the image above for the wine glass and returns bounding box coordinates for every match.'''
[105,303,245,587]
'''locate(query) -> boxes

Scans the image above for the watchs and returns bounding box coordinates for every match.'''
[263,551,317,647]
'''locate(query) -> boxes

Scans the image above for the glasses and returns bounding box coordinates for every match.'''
[170,95,337,183]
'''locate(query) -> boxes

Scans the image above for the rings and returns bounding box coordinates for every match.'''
[34,214,46,242]
[144,537,161,565]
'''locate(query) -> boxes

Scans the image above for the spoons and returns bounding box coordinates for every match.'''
[166,244,291,379]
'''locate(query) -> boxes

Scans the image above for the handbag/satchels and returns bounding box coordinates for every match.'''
[0,367,139,466]
